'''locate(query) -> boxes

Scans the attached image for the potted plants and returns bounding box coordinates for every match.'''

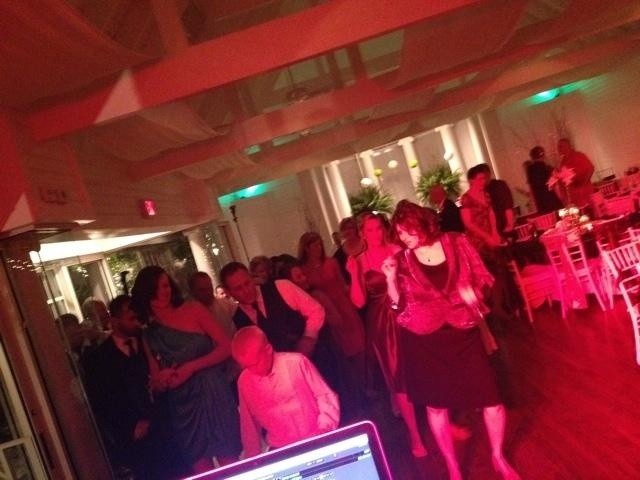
[415,162,462,207]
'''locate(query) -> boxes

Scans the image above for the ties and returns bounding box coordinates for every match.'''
[251,301,268,328]
[122,339,137,358]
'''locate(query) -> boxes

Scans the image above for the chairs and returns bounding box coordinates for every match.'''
[605,198,636,230]
[597,181,616,198]
[502,242,551,323]
[602,241,640,363]
[546,240,605,322]
[512,223,535,248]
[597,229,640,307]
[528,211,558,233]
[592,192,608,219]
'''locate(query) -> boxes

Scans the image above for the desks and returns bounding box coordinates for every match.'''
[523,215,623,309]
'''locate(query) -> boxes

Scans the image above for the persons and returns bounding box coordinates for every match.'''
[381,200,520,480]
[87,294,165,453]
[331,218,367,323]
[231,326,340,460]
[82,298,107,329]
[526,146,565,213]
[248,257,278,278]
[219,261,357,421]
[53,313,109,373]
[476,164,515,237]
[461,166,502,261]
[346,214,472,459]
[298,232,366,362]
[278,265,342,331]
[186,272,238,373]
[429,184,464,233]
[129,265,230,476]
[556,138,594,199]
